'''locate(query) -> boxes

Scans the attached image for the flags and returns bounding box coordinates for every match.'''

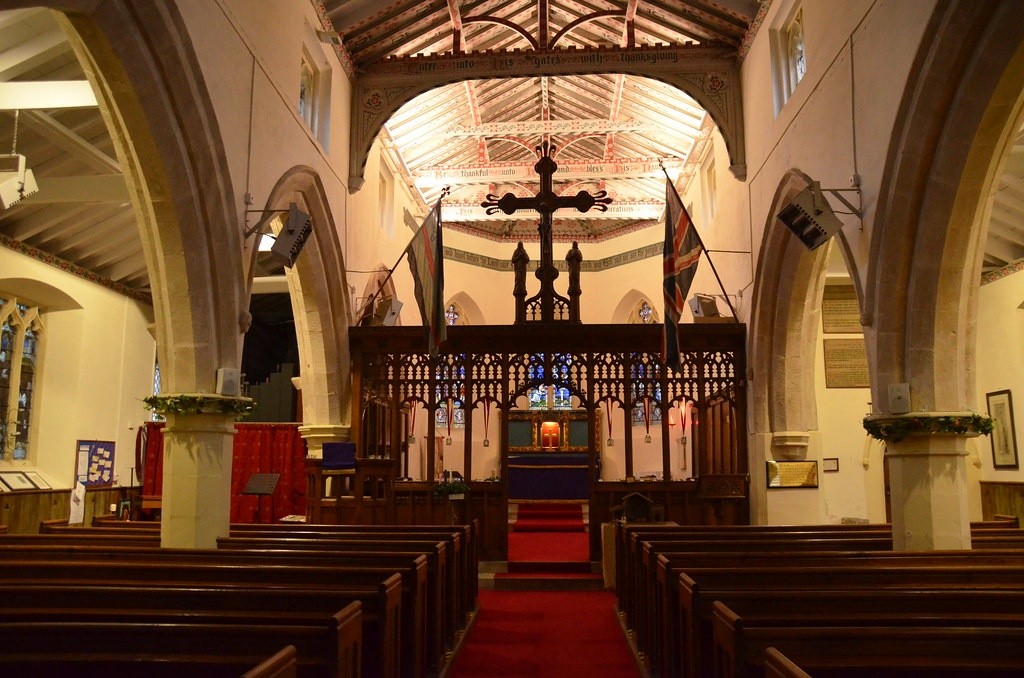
[663,182,705,375]
[408,203,447,358]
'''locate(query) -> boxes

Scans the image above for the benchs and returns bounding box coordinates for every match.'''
[0,520,482,678]
[614,513,1024,678]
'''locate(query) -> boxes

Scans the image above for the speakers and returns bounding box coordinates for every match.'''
[370,295,405,326]
[268,201,316,269]
[687,293,722,318]
[774,180,846,253]
[1,154,42,211]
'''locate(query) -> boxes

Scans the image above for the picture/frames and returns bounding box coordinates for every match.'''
[117,499,133,521]
[985,389,1019,470]
[0,471,52,492]
